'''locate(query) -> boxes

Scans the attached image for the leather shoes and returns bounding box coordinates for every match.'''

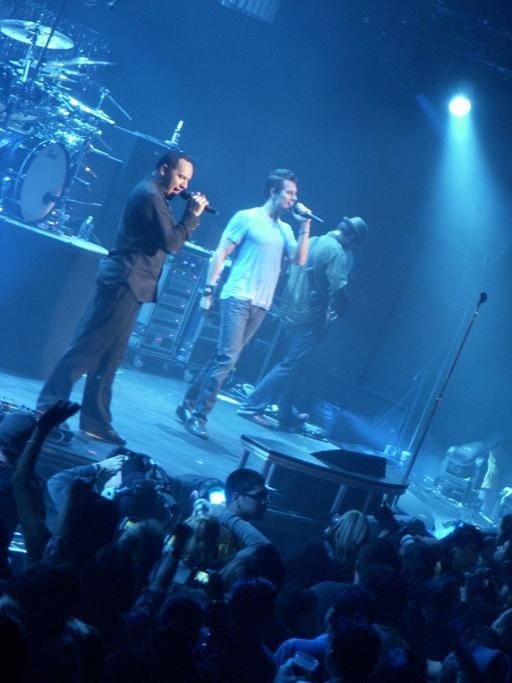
[172,404,209,441]
[237,412,273,430]
[78,428,126,449]
[278,412,310,429]
[341,215,370,245]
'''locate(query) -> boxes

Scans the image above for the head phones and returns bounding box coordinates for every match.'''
[99,479,157,501]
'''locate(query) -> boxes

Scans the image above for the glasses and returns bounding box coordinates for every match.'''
[239,490,264,502]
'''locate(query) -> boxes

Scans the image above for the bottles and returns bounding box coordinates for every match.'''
[171,120,184,147]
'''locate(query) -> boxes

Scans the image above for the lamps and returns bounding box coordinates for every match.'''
[436,441,489,513]
[169,474,226,506]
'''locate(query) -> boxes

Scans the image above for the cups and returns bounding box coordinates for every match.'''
[292,650,318,678]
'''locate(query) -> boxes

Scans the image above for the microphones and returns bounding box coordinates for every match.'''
[293,202,324,224]
[179,189,219,215]
[480,292,487,302]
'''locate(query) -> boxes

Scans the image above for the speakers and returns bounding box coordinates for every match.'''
[268,448,387,519]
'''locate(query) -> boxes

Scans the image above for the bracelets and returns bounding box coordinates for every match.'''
[204,284,216,296]
[298,229,310,236]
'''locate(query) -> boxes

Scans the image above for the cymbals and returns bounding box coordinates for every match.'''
[56,57,114,65]
[0,19,74,50]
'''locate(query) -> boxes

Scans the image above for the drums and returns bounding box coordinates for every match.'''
[0,126,70,224]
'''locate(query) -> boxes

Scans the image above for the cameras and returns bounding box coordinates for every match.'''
[183,567,216,591]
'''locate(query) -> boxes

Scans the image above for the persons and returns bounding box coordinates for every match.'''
[235,213,370,428]
[35,146,212,447]
[2,397,512,680]
[171,166,313,442]
